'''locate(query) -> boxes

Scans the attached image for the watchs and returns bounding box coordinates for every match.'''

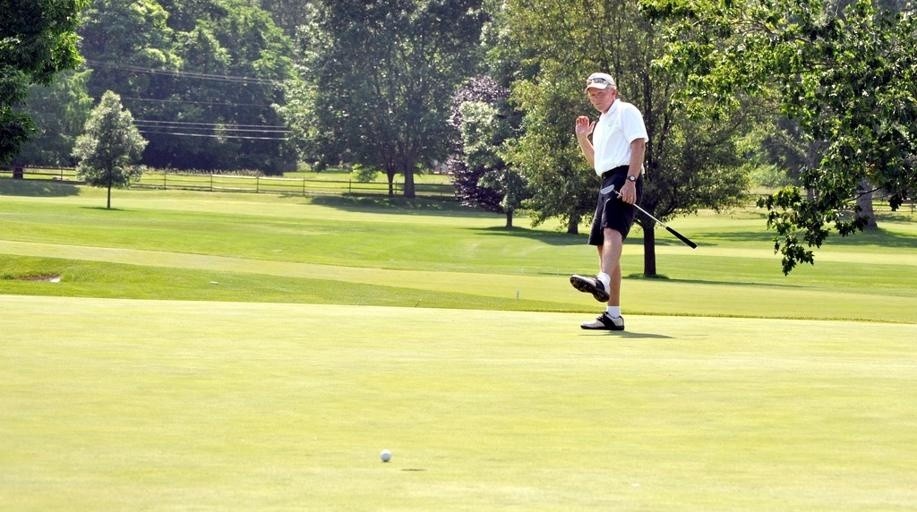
[626,175,637,182]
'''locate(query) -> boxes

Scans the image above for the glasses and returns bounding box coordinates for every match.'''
[586,78,605,84]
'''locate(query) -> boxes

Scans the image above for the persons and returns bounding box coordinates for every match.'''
[570,72,649,330]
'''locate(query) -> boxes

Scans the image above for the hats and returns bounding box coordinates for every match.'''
[584,72,616,92]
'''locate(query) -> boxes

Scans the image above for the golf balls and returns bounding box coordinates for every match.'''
[379,449,391,461]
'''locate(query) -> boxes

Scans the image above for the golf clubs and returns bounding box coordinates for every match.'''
[599,185,696,250]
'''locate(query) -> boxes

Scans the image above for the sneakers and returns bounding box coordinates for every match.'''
[581,312,624,330]
[570,276,610,303]
